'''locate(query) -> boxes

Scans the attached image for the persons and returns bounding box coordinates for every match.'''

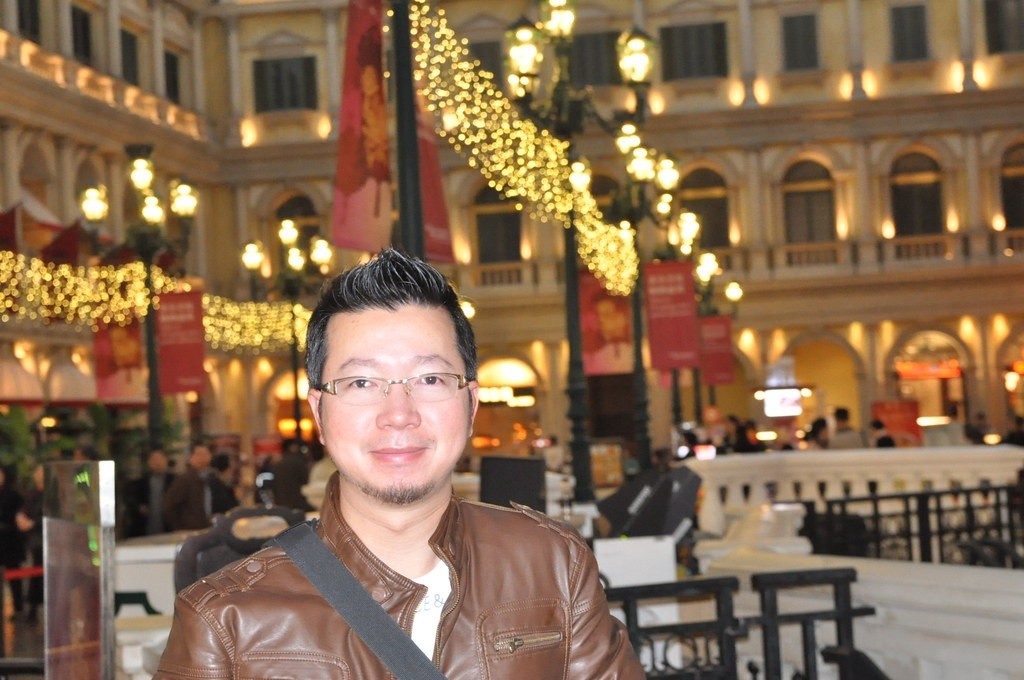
[1005,417,1024,446]
[276,440,310,510]
[155,247,644,680]
[966,415,987,444]
[0,448,101,623]
[870,420,895,447]
[678,415,766,459]
[805,409,856,449]
[123,443,241,541]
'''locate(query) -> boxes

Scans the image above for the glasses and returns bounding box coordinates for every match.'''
[317,372,472,406]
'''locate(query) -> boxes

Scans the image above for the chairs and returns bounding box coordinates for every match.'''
[174,504,305,597]
